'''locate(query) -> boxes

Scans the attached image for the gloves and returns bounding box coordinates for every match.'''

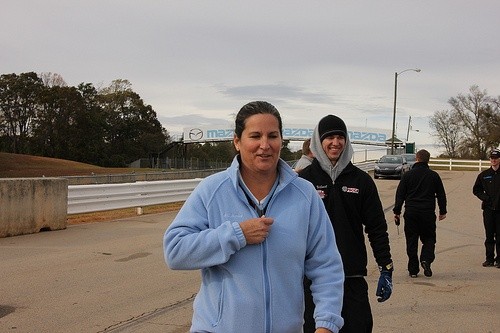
[376,271,392,302]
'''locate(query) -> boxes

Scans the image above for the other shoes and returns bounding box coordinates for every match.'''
[421,260,432,277]
[409,273,417,277]
[482,260,494,266]
[495,263,500,267]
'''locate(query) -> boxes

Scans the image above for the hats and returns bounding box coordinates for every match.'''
[489,150,500,158]
[318,114,347,142]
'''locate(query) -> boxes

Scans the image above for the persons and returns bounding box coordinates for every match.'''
[472,151,500,269]
[295,139,316,173]
[297,115,394,333]
[393,149,448,279]
[163,101,346,333]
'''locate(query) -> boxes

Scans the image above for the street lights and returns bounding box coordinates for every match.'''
[407,115,420,142]
[390,68,422,154]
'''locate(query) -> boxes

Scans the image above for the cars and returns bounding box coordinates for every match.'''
[402,153,417,169]
[374,155,411,179]
